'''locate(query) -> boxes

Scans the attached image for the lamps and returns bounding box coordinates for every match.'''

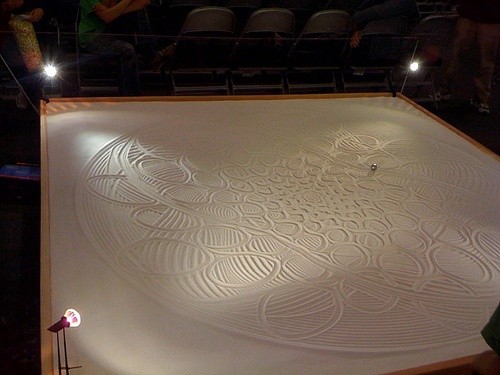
[46,309,84,375]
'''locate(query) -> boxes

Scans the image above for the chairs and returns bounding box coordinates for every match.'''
[340,16,410,92]
[390,14,459,92]
[230,7,296,95]
[75,7,120,96]
[286,10,352,94]
[167,6,237,95]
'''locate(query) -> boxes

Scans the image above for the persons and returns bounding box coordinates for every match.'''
[0,0,89,118]
[78,0,160,96]
[471,301,500,375]
[431,0,500,112]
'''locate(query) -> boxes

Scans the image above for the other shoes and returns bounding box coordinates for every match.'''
[160,42,177,58]
[471,97,490,119]
